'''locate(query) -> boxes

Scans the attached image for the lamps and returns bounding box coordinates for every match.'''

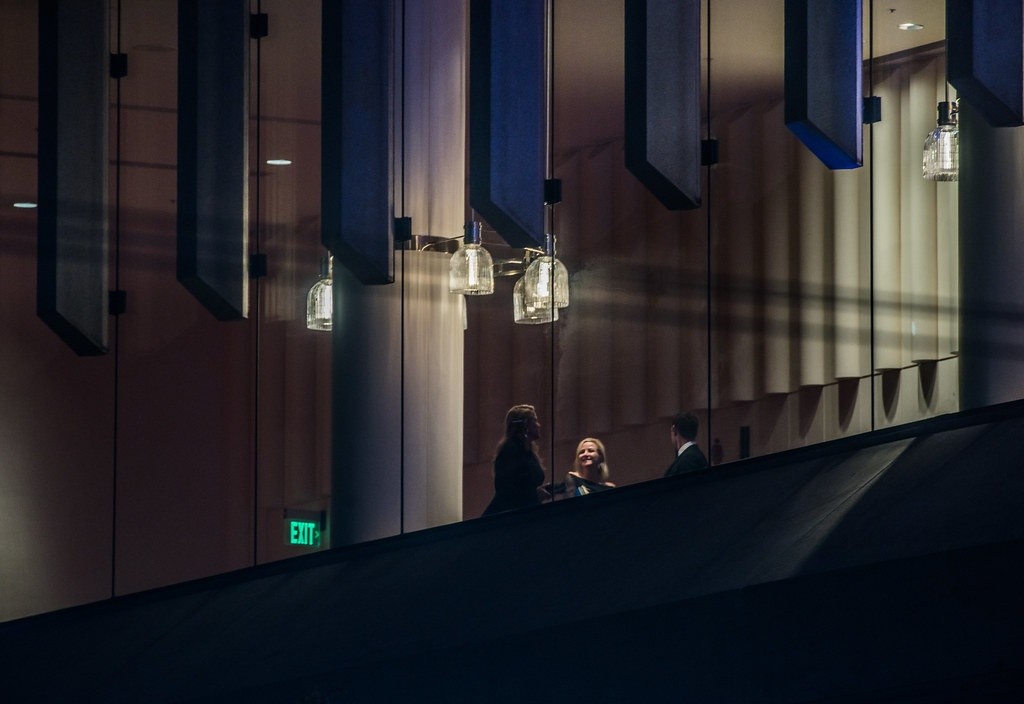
[420,210,494,295]
[922,100,958,182]
[307,256,333,331]
[525,233,569,308]
[513,275,559,323]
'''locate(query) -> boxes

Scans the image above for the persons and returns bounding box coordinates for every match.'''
[482,405,556,518]
[662,410,708,481]
[563,436,616,500]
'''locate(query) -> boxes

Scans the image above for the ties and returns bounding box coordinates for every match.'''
[674,450,679,460]
